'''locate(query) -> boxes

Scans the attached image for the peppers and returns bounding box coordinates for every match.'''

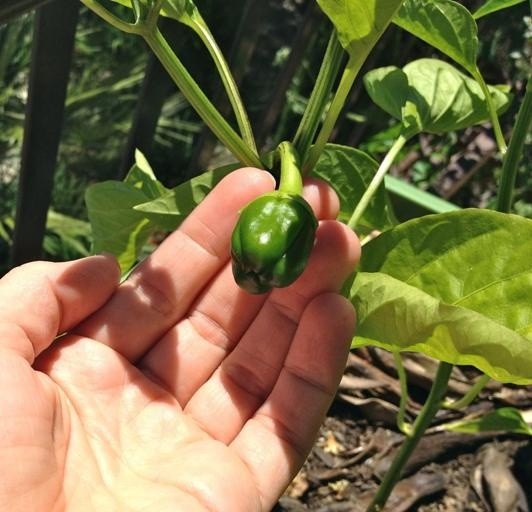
[229,194,317,296]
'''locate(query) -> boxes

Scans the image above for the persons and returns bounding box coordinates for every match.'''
[0,166,361,512]
